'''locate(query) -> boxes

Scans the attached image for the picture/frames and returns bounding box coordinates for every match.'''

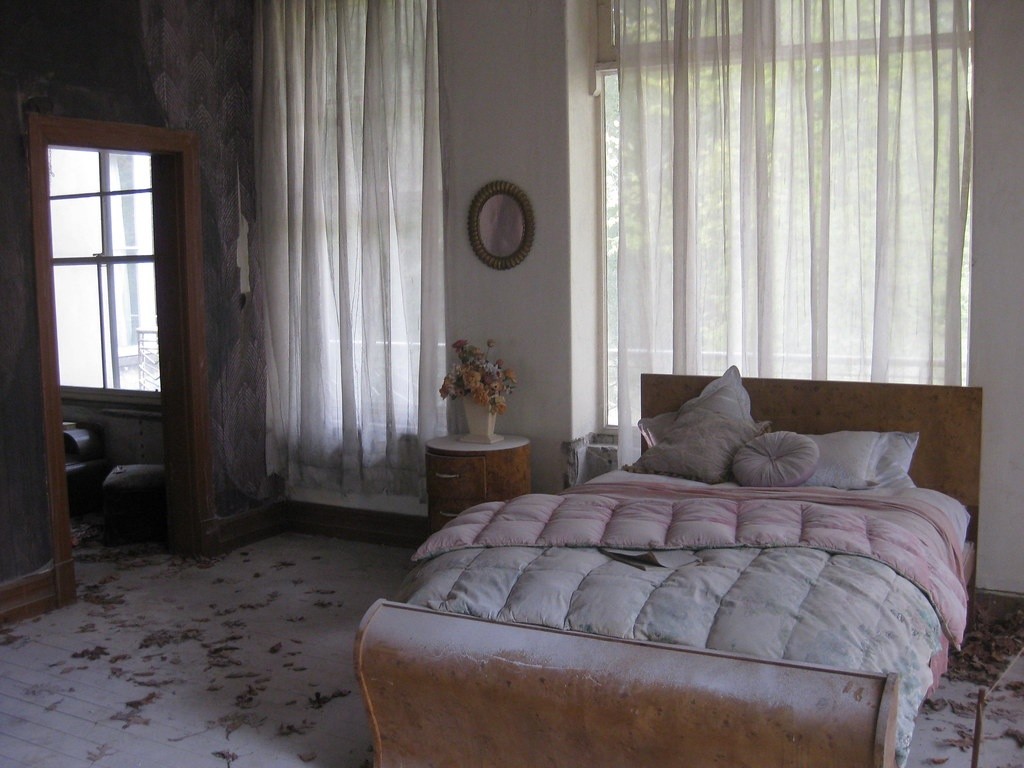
[467,180,537,270]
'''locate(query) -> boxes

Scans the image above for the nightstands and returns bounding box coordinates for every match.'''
[424,435,532,535]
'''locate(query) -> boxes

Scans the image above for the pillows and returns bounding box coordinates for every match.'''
[805,429,919,490]
[638,365,756,450]
[621,404,773,486]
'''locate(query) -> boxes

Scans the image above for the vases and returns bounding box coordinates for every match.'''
[457,396,505,445]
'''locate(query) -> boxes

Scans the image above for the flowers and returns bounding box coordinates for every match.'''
[439,337,521,416]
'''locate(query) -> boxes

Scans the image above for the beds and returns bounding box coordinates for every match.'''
[352,373,983,768]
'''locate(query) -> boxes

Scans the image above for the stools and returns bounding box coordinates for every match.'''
[101,465,167,547]
